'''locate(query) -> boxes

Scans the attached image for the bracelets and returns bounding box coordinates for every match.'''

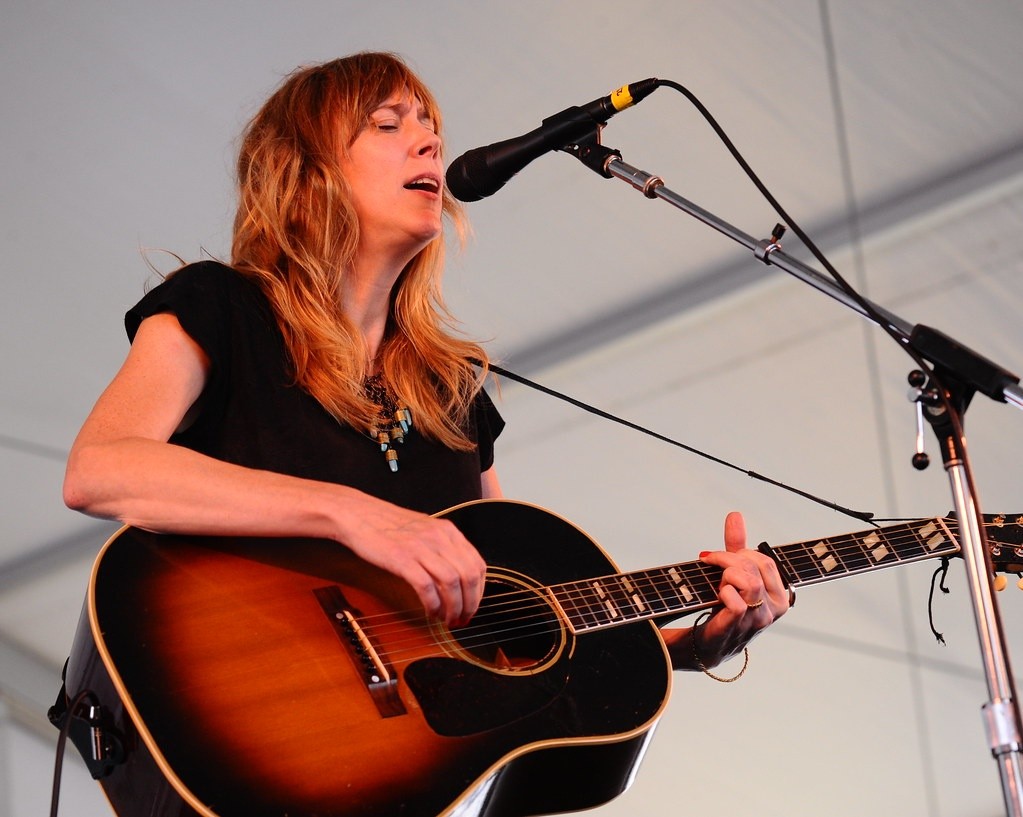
[691,612,749,682]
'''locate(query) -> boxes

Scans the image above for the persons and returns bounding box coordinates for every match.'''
[64,52,790,673]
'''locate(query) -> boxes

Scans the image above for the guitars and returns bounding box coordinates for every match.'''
[45,491,1023,817]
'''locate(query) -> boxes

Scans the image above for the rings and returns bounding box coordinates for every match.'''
[747,601,765,609]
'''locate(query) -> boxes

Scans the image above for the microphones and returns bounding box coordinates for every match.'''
[445,76,662,203]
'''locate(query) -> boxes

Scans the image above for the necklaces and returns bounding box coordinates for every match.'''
[361,373,411,471]
